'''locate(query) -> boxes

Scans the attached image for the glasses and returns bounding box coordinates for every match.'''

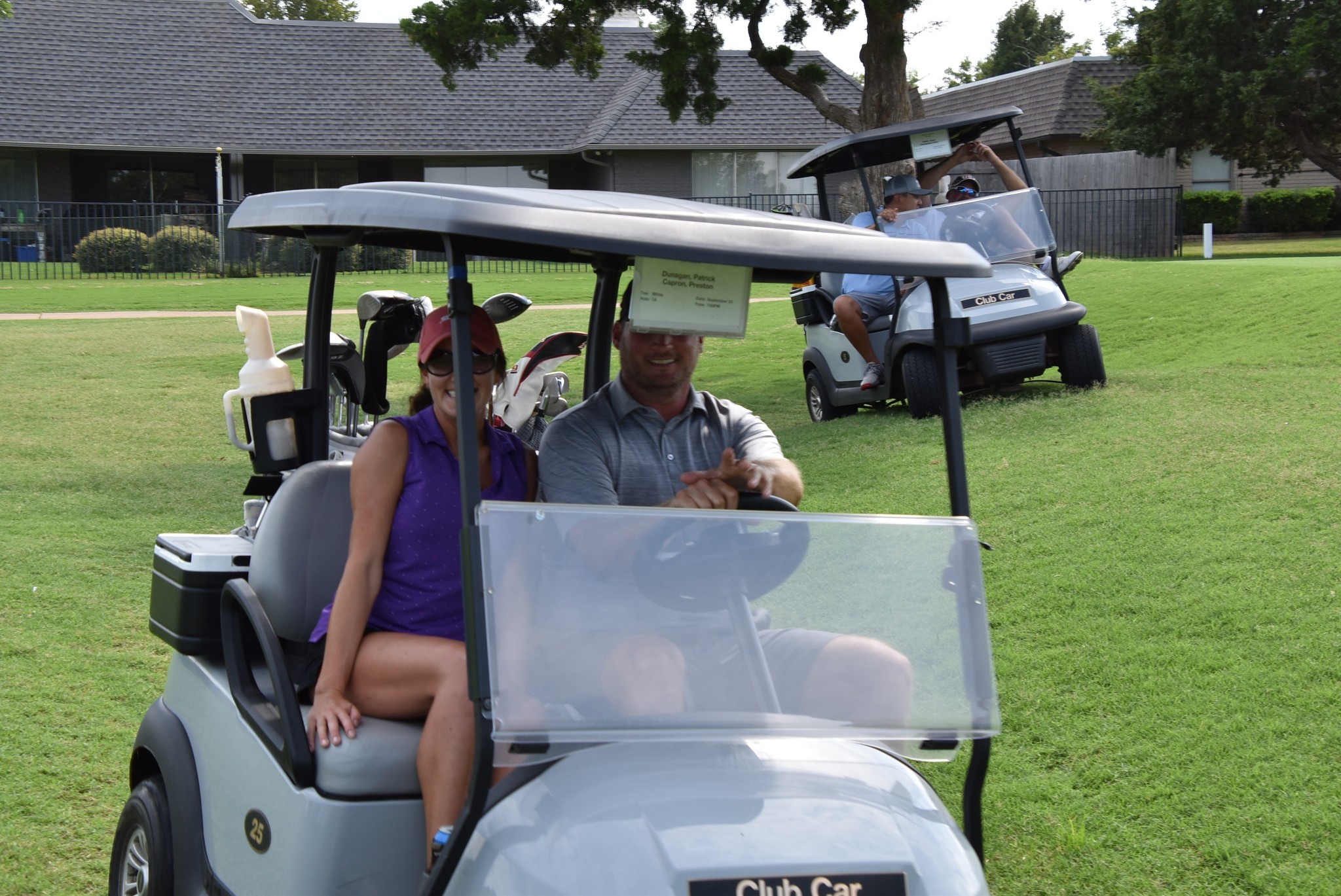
[949,186,979,198]
[425,349,497,377]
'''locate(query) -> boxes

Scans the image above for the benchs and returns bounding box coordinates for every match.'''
[249,458,424,799]
[814,271,893,332]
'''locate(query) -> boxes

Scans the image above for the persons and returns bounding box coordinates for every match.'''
[302,302,548,896]
[919,141,1084,280]
[832,176,938,388]
[537,279,916,756]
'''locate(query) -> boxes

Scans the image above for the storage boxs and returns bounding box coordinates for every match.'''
[790,284,820,325]
[16,245,39,262]
[148,532,253,657]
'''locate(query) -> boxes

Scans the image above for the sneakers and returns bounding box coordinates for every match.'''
[1040,251,1083,282]
[861,362,886,391]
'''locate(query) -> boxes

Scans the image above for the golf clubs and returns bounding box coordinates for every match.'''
[481,292,570,427]
[276,290,434,436]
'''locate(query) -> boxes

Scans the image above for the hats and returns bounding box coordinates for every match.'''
[948,174,981,194]
[884,174,934,198]
[417,305,503,363]
